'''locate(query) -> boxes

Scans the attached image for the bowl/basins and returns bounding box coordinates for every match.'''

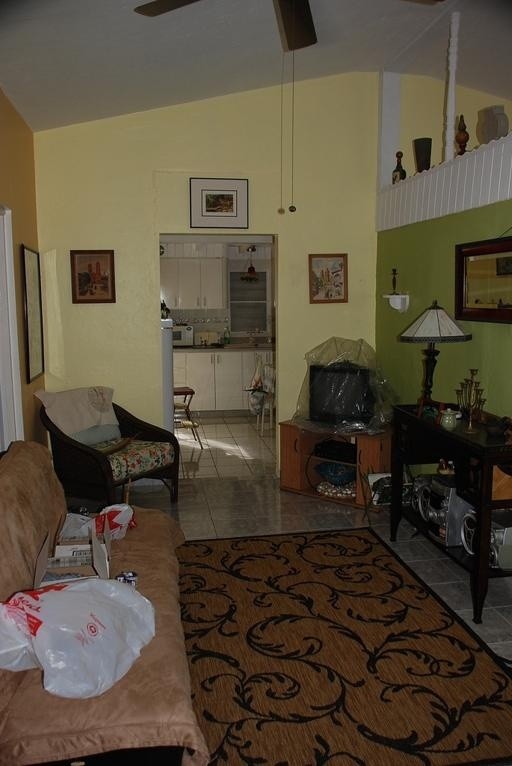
[317,461,357,486]
[485,426,504,436]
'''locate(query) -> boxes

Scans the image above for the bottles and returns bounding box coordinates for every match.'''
[438,457,456,472]
[439,407,462,431]
[200,336,207,345]
[390,149,406,185]
[224,326,231,344]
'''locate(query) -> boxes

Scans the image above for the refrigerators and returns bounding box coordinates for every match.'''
[160,318,175,437]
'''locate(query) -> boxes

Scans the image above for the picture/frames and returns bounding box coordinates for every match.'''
[454,235,512,324]
[18,243,45,383]
[187,177,249,229]
[69,249,117,303]
[307,253,348,303]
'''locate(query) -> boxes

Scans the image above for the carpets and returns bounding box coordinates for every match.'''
[179,524,512,766]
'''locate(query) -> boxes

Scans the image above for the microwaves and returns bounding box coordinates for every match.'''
[173,325,193,347]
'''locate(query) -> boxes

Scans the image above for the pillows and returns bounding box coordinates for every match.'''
[33,386,121,447]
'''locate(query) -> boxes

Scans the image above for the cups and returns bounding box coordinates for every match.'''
[413,137,434,173]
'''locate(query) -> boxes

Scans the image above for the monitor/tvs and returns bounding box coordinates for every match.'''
[309,365,374,424]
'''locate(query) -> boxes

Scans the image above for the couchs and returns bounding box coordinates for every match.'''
[3,437,209,765]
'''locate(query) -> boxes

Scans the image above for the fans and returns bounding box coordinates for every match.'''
[132,0,449,52]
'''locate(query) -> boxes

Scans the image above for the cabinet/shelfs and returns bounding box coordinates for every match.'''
[159,241,228,310]
[356,433,382,511]
[392,398,512,624]
[225,244,273,343]
[185,351,244,410]
[244,350,276,411]
[278,418,301,493]
[301,426,357,504]
[171,352,184,412]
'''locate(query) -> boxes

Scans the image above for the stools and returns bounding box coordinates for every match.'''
[172,386,204,450]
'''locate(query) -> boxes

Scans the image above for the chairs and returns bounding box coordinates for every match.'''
[255,365,276,438]
[39,385,179,514]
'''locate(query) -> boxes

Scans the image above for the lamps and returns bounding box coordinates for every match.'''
[239,243,260,280]
[397,299,473,421]
